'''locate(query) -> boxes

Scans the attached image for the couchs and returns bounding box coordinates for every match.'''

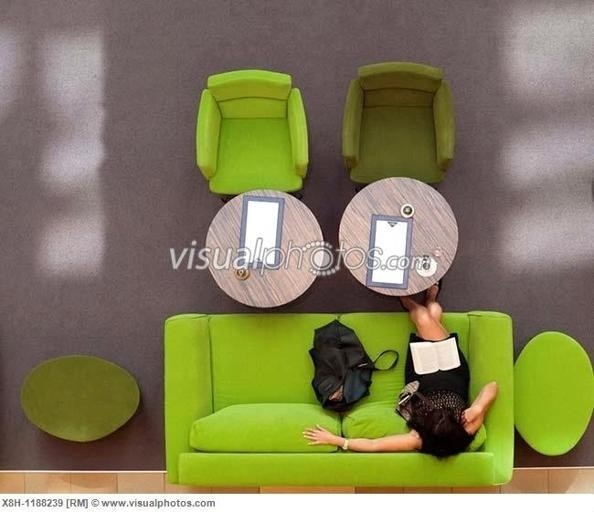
[163,310,514,487]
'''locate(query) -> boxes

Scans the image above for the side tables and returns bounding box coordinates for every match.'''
[512,329,594,458]
[18,354,142,443]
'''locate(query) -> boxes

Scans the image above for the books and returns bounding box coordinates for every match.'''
[408,338,462,375]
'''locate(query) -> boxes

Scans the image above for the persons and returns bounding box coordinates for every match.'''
[302,279,498,460]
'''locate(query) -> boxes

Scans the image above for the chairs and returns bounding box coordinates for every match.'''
[341,62,455,189]
[196,70,310,204]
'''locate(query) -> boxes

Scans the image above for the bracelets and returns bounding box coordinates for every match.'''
[342,439,348,451]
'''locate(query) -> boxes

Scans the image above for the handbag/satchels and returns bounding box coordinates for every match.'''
[308,319,398,412]
[395,381,420,423]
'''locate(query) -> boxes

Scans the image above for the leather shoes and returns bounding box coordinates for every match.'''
[426,279,442,302]
